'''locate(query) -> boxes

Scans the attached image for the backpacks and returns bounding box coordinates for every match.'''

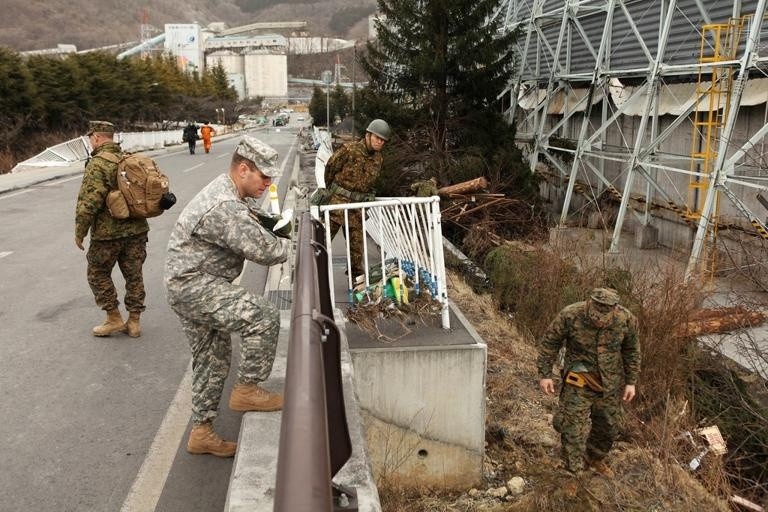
[93,150,169,218]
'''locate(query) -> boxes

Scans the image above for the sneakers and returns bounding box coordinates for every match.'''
[591,460,614,479]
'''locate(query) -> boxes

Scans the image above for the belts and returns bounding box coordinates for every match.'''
[333,183,365,201]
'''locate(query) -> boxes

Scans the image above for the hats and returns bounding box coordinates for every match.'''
[237,135,282,177]
[589,287,620,328]
[85,121,114,135]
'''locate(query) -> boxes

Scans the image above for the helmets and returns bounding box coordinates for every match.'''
[366,119,390,140]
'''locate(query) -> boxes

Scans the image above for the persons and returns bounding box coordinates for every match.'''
[314,118,391,279]
[198,119,214,154]
[162,132,291,460]
[534,286,642,481]
[73,118,149,338]
[182,120,200,154]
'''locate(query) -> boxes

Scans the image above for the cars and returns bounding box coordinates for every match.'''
[273,108,293,126]
[297,115,304,121]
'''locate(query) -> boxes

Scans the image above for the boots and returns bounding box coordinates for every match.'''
[92,308,126,336]
[187,422,236,457]
[123,312,141,337]
[230,382,284,412]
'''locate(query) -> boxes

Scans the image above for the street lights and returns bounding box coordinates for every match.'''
[132,82,158,134]
[215,108,225,126]
